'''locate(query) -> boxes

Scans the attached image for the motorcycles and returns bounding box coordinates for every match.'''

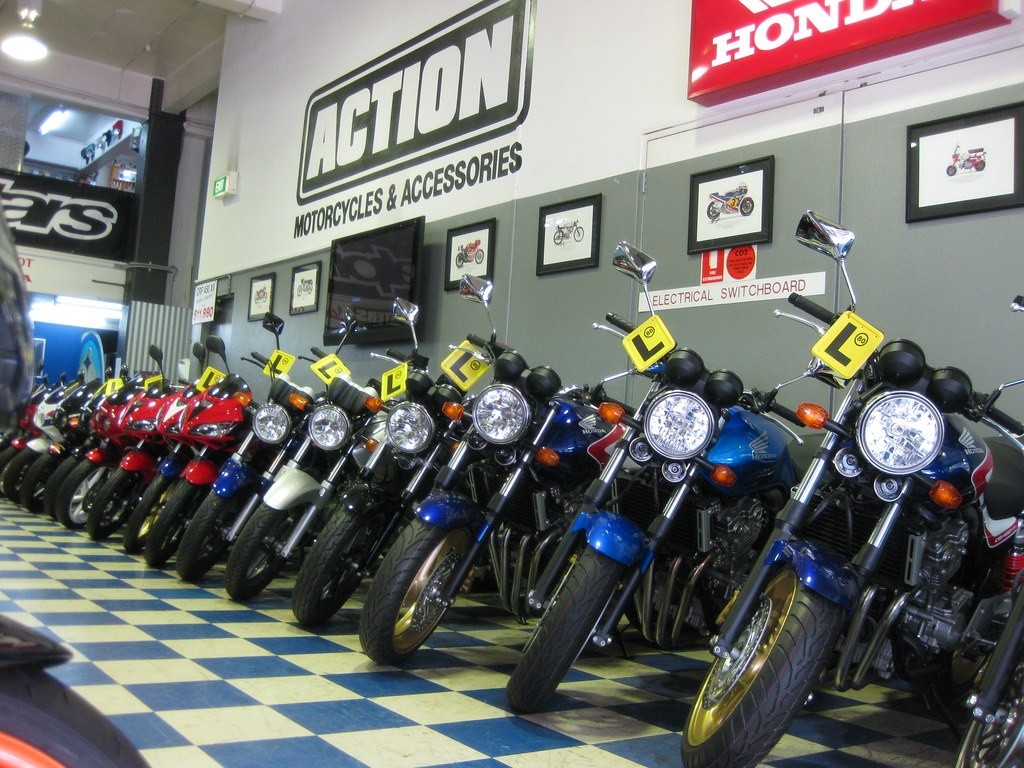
[1,212,1024,768]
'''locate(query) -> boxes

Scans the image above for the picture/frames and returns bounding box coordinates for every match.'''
[687,156,775,254]
[905,102,1024,222]
[443,217,496,291]
[536,192,602,276]
[289,260,323,316]
[247,271,277,322]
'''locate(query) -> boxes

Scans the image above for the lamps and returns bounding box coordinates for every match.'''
[1,0,51,64]
